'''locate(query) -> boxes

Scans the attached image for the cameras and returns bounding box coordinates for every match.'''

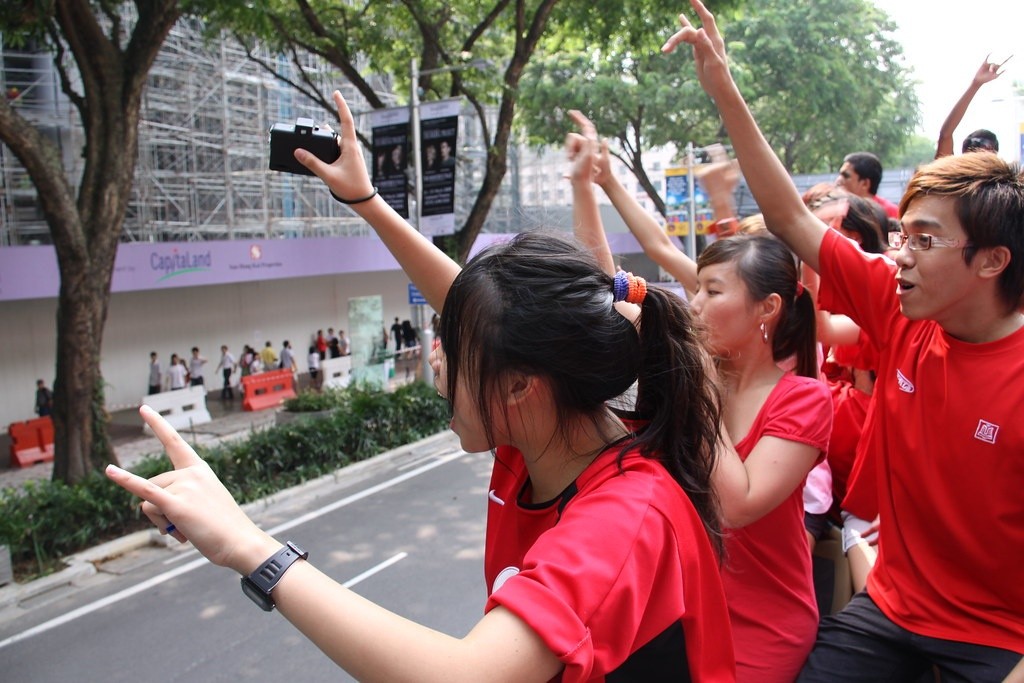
[268,117,341,175]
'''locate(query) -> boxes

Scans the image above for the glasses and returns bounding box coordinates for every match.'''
[888,231,978,250]
[964,137,992,149]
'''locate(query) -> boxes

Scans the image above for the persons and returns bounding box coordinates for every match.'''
[167,353,188,390]
[430,313,437,330]
[317,329,328,361]
[326,328,352,359]
[400,320,421,361]
[369,317,403,358]
[148,351,162,395]
[239,344,263,399]
[105,87,732,683]
[281,341,299,395]
[261,341,278,371]
[188,347,208,386]
[563,0,1024,683]
[180,360,190,386]
[214,345,238,400]
[306,346,320,390]
[35,379,53,417]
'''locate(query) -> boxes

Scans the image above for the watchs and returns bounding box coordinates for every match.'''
[240,539,308,611]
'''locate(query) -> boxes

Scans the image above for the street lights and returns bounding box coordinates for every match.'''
[409,51,488,384]
